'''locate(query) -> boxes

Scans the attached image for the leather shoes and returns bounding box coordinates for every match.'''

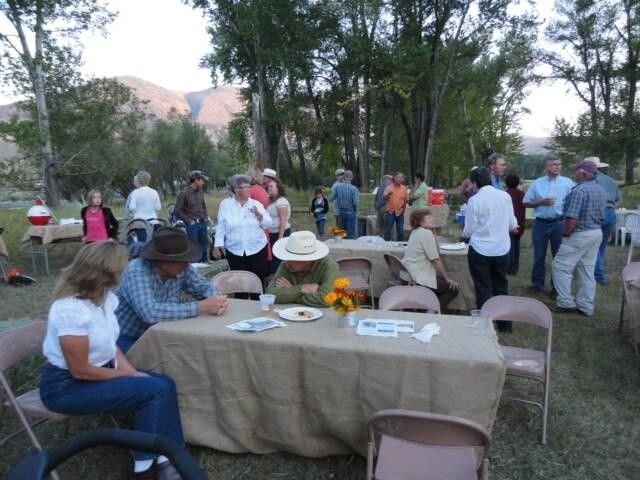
[156,460,180,480]
[550,305,577,313]
[132,465,156,479]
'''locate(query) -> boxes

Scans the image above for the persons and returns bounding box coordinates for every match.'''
[399,206,460,313]
[332,168,345,226]
[38,237,187,479]
[328,168,359,239]
[212,172,272,302]
[111,225,230,354]
[310,188,329,234]
[374,174,394,237]
[264,230,341,308]
[504,172,527,276]
[461,163,519,335]
[552,159,608,317]
[174,169,210,262]
[80,188,119,245]
[383,170,408,241]
[265,178,291,275]
[522,155,577,298]
[459,164,481,203]
[262,167,278,190]
[410,173,428,209]
[250,178,273,274]
[125,170,162,223]
[584,156,624,287]
[487,151,506,192]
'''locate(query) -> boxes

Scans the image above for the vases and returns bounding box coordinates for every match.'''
[335,236,343,243]
[336,311,358,328]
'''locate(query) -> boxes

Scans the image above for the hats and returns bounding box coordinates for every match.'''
[262,167,278,179]
[569,160,599,173]
[140,226,203,261]
[335,168,344,176]
[189,170,210,181]
[272,230,329,261]
[585,156,610,168]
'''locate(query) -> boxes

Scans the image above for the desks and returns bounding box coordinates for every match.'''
[322,238,469,317]
[149,298,506,456]
[28,217,126,278]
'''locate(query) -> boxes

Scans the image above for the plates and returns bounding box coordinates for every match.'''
[278,307,324,322]
[438,242,467,251]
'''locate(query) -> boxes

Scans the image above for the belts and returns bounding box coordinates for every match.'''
[536,217,563,224]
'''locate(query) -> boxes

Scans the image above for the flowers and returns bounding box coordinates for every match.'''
[327,226,347,238]
[324,277,364,313]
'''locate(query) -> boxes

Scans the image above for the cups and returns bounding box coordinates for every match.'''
[470,310,491,334]
[259,294,276,319]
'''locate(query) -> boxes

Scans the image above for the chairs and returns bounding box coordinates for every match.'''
[209,270,263,302]
[367,410,492,480]
[378,285,441,315]
[620,213,640,265]
[289,194,311,217]
[618,229,640,332]
[336,258,374,310]
[382,252,422,286]
[479,295,552,446]
[0,321,141,480]
